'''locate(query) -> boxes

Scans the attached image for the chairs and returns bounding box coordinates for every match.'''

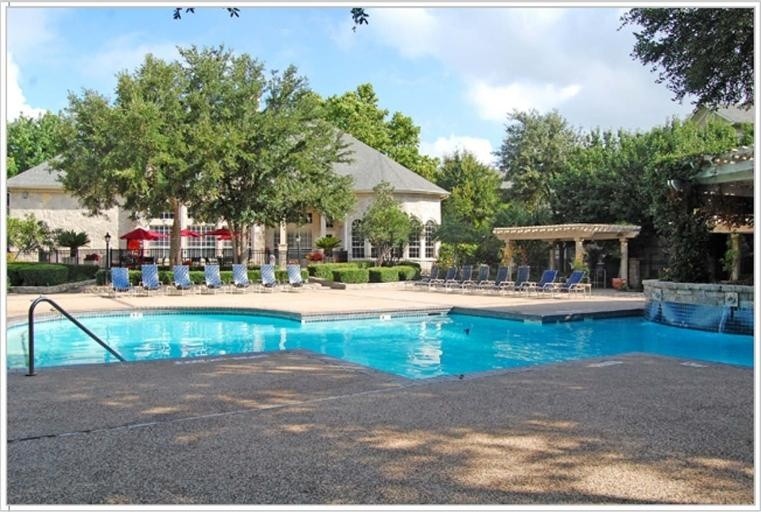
[109,261,310,294]
[415,265,593,300]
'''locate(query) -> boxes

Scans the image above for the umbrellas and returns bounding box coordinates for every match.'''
[179,229,203,261]
[127,239,140,264]
[149,230,168,240]
[205,226,240,264]
[218,235,232,240]
[119,228,159,257]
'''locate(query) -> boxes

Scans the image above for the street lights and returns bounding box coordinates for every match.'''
[105,233,110,270]
